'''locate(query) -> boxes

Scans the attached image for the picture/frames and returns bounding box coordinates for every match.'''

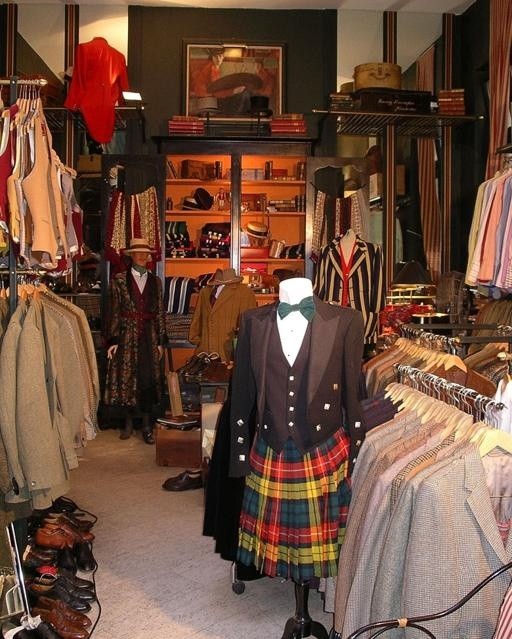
[178,38,286,127]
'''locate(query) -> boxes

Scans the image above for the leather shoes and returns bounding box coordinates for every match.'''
[15,497,95,639]
[144,432,155,444]
[45,276,102,293]
[163,470,202,491]
[177,356,233,382]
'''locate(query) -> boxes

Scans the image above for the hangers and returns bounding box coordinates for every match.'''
[3,79,45,133]
[0,269,85,343]
[482,290,512,315]
[475,153,512,191]
[359,324,512,488]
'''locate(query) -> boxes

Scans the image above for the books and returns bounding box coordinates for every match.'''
[328,89,358,111]
[268,113,308,136]
[438,87,466,116]
[167,116,205,137]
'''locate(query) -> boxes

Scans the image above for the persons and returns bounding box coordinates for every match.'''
[192,48,246,102]
[310,226,387,349]
[225,275,367,580]
[185,264,264,368]
[251,56,276,97]
[105,236,167,446]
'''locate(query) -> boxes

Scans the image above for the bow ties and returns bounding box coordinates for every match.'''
[278,296,315,323]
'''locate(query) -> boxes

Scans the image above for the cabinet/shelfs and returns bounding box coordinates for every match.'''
[151,134,315,315]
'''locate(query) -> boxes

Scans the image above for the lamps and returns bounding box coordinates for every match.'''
[386,256,433,308]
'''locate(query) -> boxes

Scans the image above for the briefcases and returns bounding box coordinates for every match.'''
[353,87,431,115]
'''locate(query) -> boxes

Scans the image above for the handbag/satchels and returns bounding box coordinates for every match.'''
[77,154,102,173]
[264,275,279,292]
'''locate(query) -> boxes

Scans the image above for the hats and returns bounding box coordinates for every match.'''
[121,238,157,256]
[205,48,224,55]
[246,96,273,118]
[184,187,212,210]
[206,267,244,285]
[195,97,221,114]
[242,221,269,239]
[497,127,512,153]
